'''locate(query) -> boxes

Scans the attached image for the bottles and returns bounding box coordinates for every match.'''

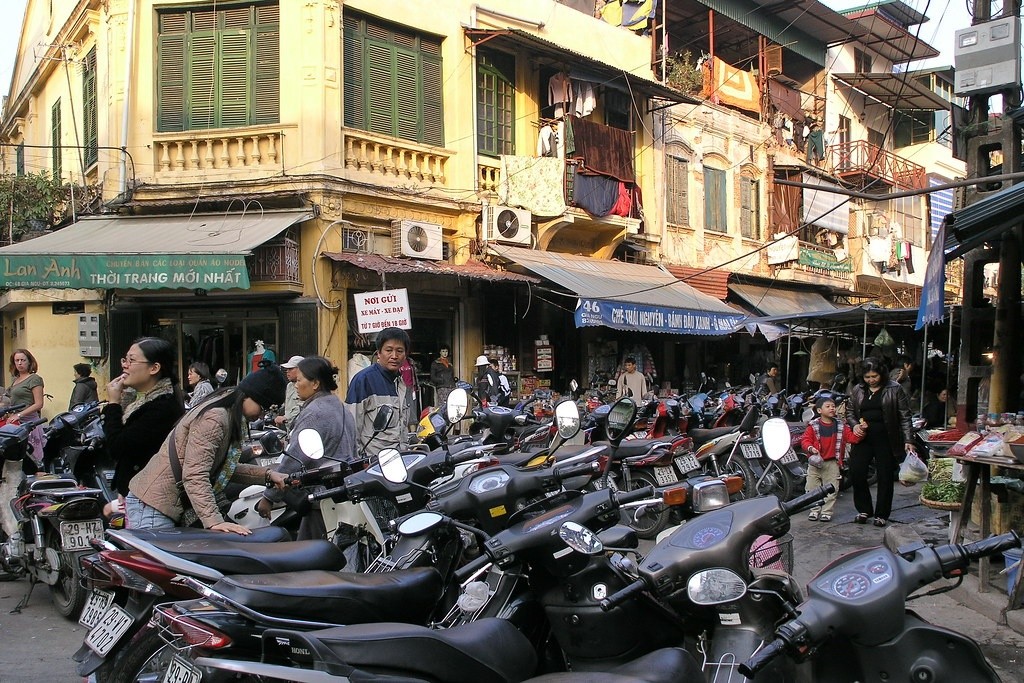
[976,411,1024,433]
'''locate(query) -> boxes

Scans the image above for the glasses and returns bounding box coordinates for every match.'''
[260,405,269,415]
[121,358,148,366]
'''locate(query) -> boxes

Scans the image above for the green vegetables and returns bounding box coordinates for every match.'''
[923,458,969,504]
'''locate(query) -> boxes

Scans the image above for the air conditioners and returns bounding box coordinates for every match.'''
[390,219,443,261]
[481,205,531,248]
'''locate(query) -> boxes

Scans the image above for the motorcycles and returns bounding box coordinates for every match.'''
[0,372,1024,682]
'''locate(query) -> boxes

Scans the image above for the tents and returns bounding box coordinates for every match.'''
[740,297,964,413]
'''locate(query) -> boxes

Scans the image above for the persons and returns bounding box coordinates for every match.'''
[188,362,214,408]
[101,327,410,536]
[258,356,359,541]
[247,339,276,375]
[923,387,950,430]
[431,344,459,435]
[343,327,410,458]
[473,355,511,408]
[890,355,913,395]
[101,336,185,497]
[615,358,658,406]
[281,356,305,435]
[4,349,44,475]
[801,397,868,522]
[125,359,289,536]
[754,362,778,396]
[68,363,101,416]
[845,358,916,527]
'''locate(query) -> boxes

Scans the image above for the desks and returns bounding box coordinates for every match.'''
[915,429,1024,595]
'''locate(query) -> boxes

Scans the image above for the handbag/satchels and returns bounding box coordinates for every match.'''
[898,451,928,487]
[808,452,824,469]
[181,491,232,529]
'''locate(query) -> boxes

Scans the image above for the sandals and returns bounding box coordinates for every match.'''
[808,512,818,520]
[820,515,832,521]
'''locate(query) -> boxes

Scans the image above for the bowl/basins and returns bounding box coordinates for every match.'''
[1008,443,1024,464]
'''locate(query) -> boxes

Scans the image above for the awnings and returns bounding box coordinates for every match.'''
[0,208,317,290]
[915,181,1024,329]
[487,242,855,341]
[462,0,951,111]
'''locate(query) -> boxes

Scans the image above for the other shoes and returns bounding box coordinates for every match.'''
[855,513,868,524]
[873,517,886,526]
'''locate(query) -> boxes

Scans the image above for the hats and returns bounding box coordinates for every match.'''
[280,356,305,368]
[474,355,490,367]
[239,364,287,410]
[73,363,92,377]
